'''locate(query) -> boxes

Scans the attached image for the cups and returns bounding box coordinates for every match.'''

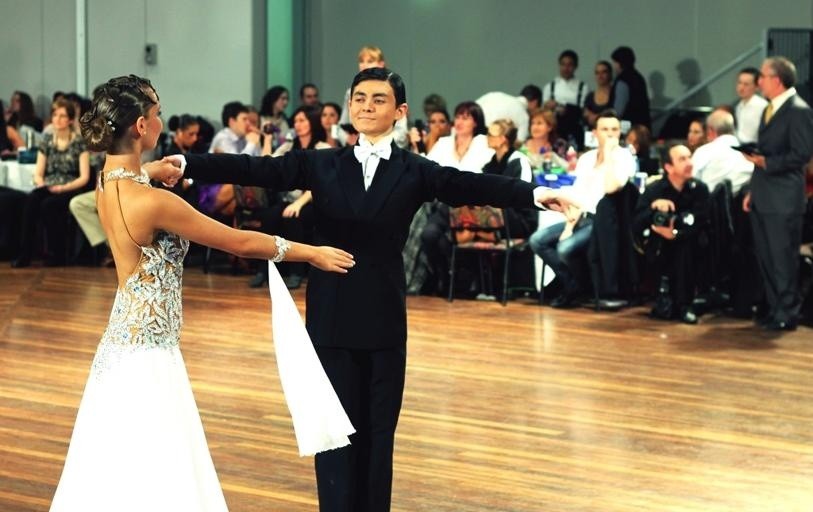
[635,172,647,194]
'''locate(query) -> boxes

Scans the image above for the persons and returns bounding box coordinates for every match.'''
[162,84,342,287]
[738,66,771,144]
[403,50,653,309]
[47,75,357,512]
[336,49,409,150]
[0,91,114,268]
[158,65,583,512]
[624,110,753,323]
[743,56,812,328]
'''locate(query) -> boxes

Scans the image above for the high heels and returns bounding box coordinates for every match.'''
[284,271,304,291]
[248,268,270,288]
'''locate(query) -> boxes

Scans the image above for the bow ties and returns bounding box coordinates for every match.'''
[351,138,393,162]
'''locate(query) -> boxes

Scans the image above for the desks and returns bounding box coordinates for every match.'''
[2,161,44,192]
[533,211,563,290]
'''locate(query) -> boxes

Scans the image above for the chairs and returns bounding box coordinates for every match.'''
[448,205,523,307]
[205,211,241,276]
[540,254,599,314]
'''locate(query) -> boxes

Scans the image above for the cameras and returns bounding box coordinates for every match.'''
[652,210,682,228]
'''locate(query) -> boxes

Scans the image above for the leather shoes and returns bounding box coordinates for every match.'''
[756,317,794,330]
[549,290,593,308]
[673,306,698,323]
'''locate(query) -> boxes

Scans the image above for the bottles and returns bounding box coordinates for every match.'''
[537,148,552,173]
[566,145,578,176]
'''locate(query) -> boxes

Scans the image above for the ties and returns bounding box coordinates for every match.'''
[765,104,775,124]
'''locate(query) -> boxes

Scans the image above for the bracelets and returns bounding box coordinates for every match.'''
[271,236,288,263]
